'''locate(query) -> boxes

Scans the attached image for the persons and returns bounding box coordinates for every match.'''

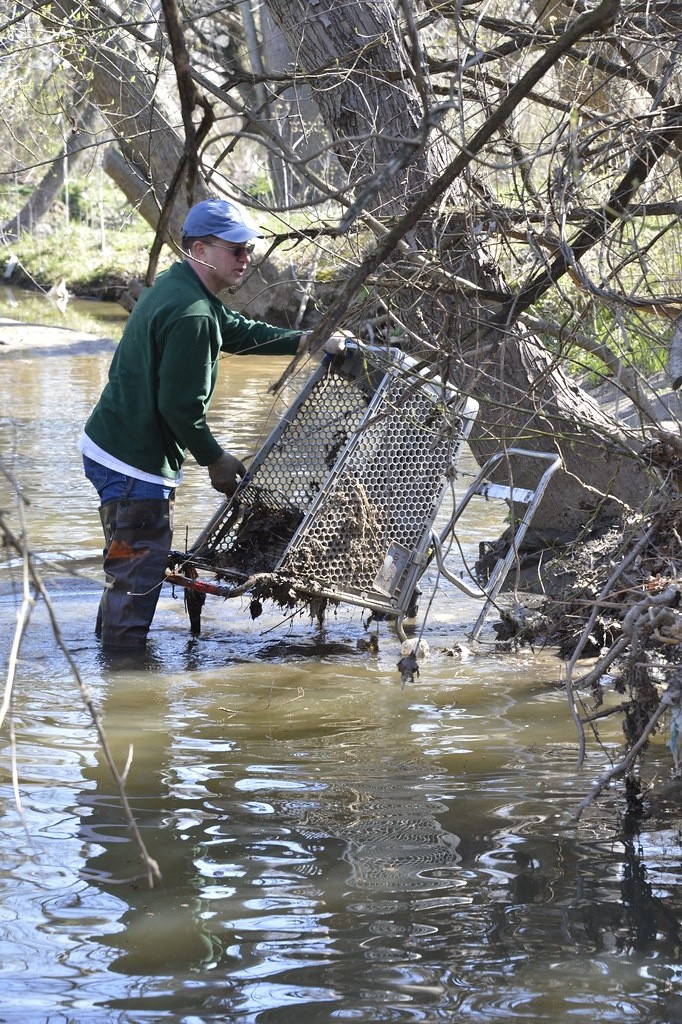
[78,198,366,651]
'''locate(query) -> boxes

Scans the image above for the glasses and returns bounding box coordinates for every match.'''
[202,241,256,257]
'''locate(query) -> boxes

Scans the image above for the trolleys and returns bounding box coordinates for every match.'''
[159,338,564,659]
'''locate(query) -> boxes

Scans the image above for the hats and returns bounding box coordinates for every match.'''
[182,199,264,243]
